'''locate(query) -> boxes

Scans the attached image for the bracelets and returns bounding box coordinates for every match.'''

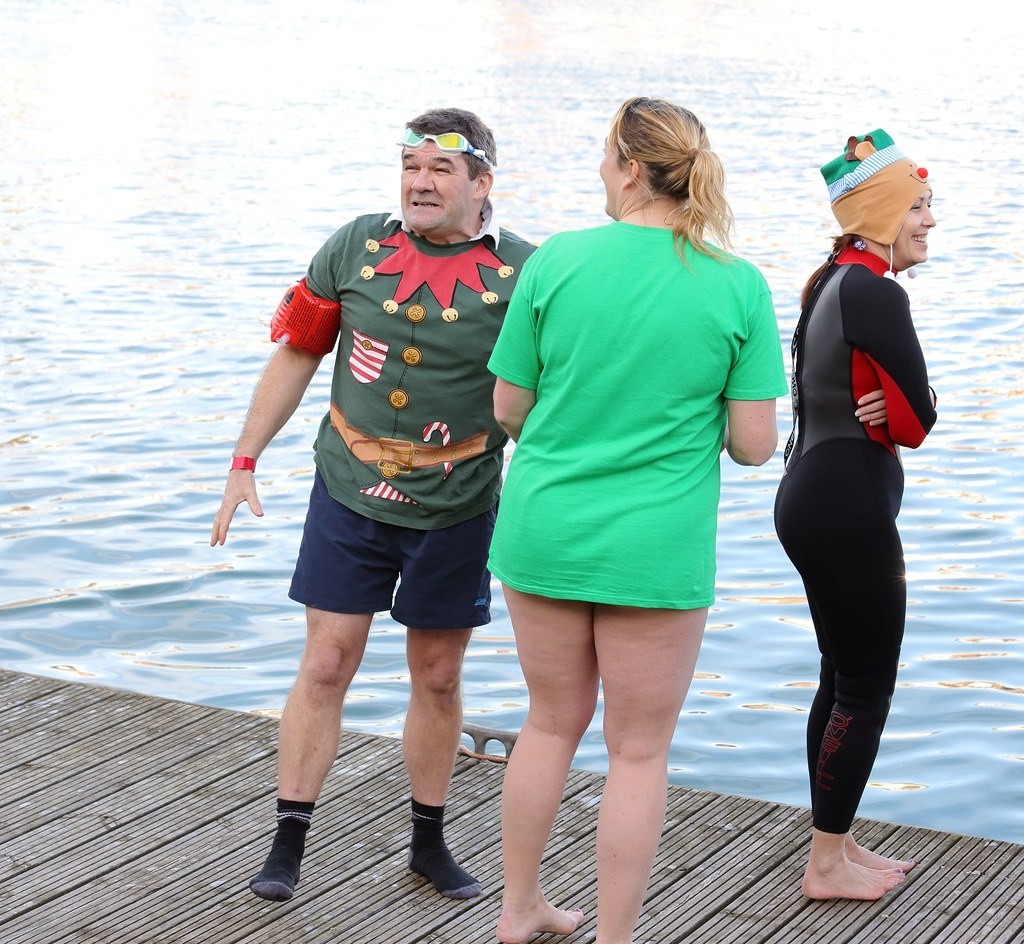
[228,456,257,474]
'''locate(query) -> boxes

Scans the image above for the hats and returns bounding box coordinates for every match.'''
[819,128,932,281]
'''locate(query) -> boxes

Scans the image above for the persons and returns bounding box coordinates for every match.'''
[211,109,539,901]
[775,129,936,900]
[485,97,790,944]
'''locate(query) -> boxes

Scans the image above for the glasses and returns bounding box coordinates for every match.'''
[399,126,496,168]
